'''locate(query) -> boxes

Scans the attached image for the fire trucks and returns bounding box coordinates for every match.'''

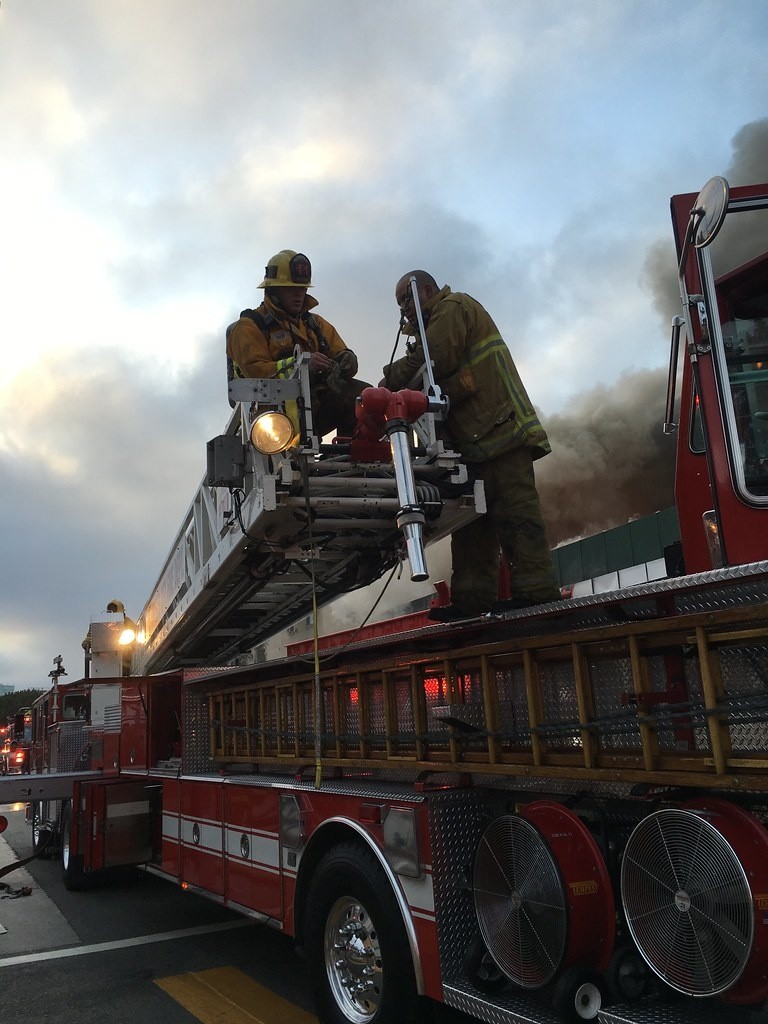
[0,174,768,1023]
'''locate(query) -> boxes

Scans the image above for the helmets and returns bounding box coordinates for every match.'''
[257,250,314,287]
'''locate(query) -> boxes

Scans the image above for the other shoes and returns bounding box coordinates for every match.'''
[428,604,467,622]
[494,596,543,610]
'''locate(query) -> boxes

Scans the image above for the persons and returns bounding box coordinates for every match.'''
[376,270,564,610]
[82,599,137,676]
[226,249,374,444]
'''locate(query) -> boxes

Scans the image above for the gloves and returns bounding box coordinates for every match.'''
[324,350,358,392]
